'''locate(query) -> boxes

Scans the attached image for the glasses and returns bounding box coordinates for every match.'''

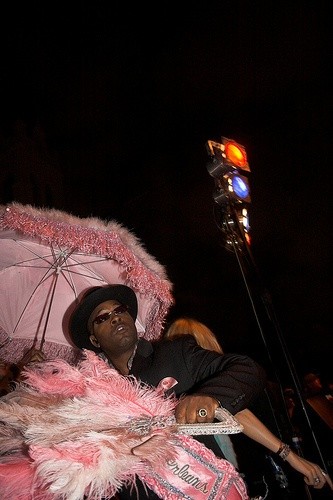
[91,305,130,337]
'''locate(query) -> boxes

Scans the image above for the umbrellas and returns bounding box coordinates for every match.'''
[0,202,174,372]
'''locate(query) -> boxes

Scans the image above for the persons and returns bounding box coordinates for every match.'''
[0,349,47,401]
[164,318,333,500]
[69,284,262,500]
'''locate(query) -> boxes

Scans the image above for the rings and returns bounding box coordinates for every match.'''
[321,470,328,477]
[314,477,320,483]
[197,409,207,418]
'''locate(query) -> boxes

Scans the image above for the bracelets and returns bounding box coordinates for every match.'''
[276,441,291,461]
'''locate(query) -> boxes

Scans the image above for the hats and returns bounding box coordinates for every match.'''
[67,284,138,352]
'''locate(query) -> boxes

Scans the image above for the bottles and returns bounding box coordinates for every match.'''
[264,451,291,495]
